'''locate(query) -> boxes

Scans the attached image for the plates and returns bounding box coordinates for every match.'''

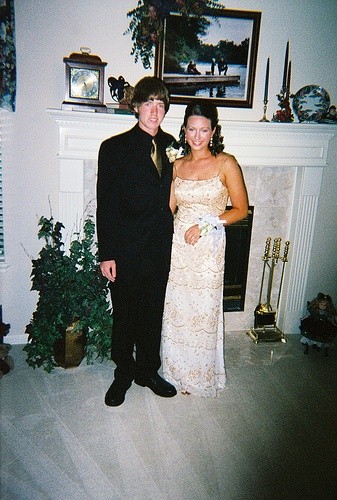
[294,85,330,121]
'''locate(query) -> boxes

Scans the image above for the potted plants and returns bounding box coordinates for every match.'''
[19,191,112,373]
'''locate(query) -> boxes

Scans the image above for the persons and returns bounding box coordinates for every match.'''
[187,61,201,75]
[96,76,177,408]
[218,57,228,75]
[211,58,216,75]
[160,102,248,398]
[300,292,337,356]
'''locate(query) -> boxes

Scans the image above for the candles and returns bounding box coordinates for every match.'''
[264,57,270,101]
[283,41,291,93]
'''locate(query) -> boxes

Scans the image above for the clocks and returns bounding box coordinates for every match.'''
[61,47,107,112]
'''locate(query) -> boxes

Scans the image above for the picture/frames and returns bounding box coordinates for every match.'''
[154,10,262,109]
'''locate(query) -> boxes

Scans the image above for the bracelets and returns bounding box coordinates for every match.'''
[196,214,227,236]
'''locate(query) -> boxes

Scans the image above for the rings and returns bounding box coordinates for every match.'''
[192,237,194,238]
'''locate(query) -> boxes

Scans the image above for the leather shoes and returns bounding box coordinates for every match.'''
[134,370,176,398]
[105,376,134,407]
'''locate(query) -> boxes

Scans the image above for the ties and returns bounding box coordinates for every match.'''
[150,138,162,177]
